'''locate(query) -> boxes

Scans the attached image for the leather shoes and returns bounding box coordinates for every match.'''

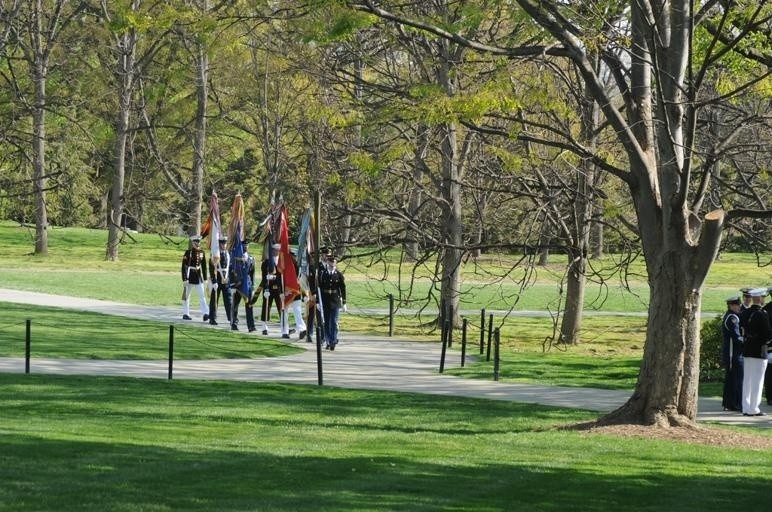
[182,314,338,350]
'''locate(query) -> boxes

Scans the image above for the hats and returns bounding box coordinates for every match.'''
[189,235,337,261]
[739,287,754,293]
[726,296,741,305]
[748,287,768,297]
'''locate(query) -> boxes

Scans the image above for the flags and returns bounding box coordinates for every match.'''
[202,190,318,311]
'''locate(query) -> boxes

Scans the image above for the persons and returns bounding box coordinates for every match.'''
[182,235,211,321]
[320,256,347,351]
[207,238,332,342]
[723,286,772,417]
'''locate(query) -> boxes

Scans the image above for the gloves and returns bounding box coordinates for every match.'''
[183,274,348,313]
